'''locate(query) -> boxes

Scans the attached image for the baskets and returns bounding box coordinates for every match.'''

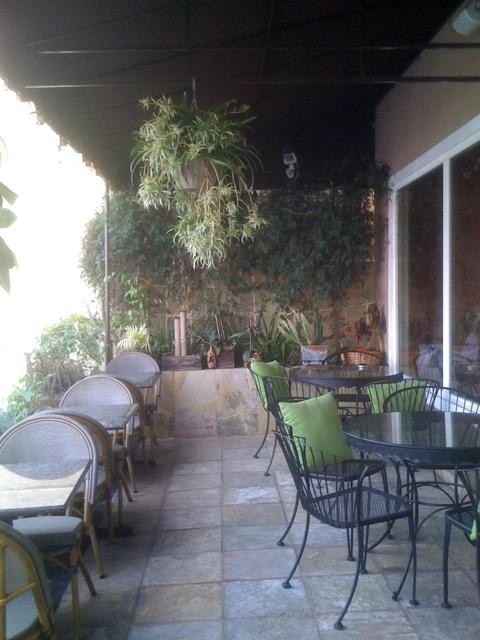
[345,334,384,366]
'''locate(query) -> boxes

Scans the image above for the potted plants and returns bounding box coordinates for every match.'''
[128,88,273,271]
[186,308,278,369]
